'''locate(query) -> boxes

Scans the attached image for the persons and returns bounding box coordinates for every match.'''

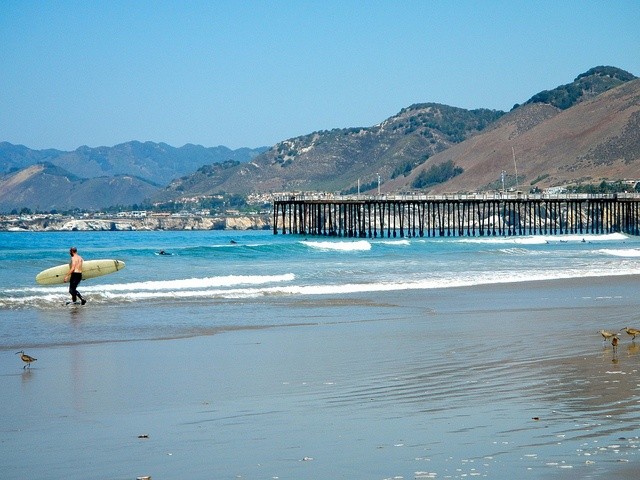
[64,247,86,305]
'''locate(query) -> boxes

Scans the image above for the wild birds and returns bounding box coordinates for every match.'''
[600,328,617,342]
[15,350,37,368]
[620,326,640,340]
[611,334,620,350]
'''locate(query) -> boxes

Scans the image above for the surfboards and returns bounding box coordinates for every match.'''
[36,260,126,293]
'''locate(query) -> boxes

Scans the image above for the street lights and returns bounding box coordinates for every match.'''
[375,173,381,196]
[501,169,506,199]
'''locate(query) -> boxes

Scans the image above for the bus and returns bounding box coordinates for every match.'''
[131,211,146,217]
